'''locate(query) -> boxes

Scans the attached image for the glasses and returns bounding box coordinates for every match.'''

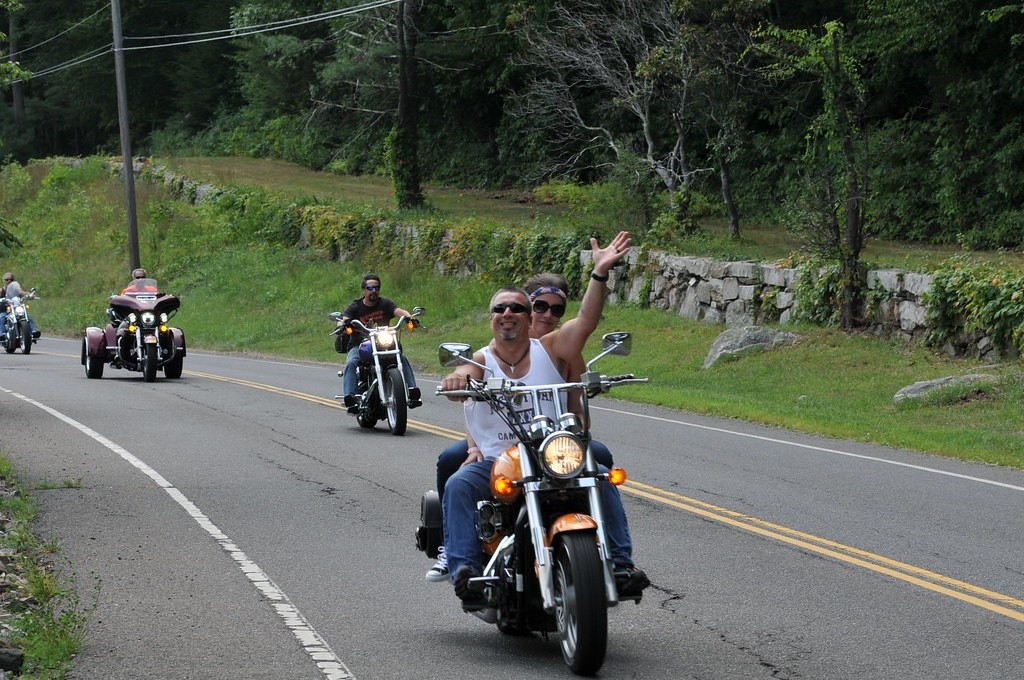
[135,276,145,279]
[491,303,530,318]
[364,285,380,291]
[4,279,13,281]
[532,299,565,317]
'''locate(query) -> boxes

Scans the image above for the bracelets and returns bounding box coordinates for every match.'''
[592,272,609,282]
[467,447,479,454]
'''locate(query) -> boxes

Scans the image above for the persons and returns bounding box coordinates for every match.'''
[337,274,422,414]
[425,231,651,612]
[0,272,41,342]
[112,268,164,363]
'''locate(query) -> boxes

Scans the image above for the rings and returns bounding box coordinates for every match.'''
[617,250,620,253]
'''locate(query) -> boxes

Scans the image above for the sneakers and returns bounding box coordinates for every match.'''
[426,546,451,582]
[454,566,483,612]
[614,567,650,602]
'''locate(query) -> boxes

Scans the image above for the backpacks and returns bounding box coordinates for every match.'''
[335,332,350,353]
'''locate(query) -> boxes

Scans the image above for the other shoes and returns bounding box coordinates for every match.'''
[0,335,6,346]
[32,331,41,341]
[343,395,360,414]
[409,387,421,409]
[114,355,122,370]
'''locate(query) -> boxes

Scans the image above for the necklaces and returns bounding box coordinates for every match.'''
[492,341,530,374]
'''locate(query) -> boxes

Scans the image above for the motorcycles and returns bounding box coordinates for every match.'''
[81,279,186,383]
[328,306,425,436]
[0,281,40,354]
[414,331,643,677]
